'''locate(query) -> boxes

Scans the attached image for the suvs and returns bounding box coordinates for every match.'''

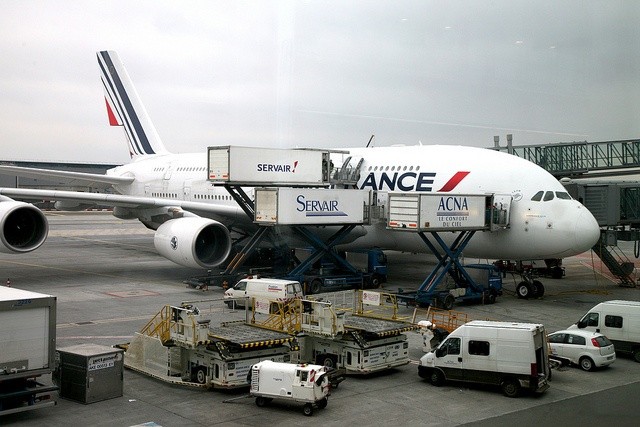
[547,329,616,370]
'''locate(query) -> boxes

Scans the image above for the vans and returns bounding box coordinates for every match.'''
[225,277,301,314]
[569,300,636,361]
[419,320,550,397]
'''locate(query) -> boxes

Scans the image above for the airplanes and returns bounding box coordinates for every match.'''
[0,51,603,300]
